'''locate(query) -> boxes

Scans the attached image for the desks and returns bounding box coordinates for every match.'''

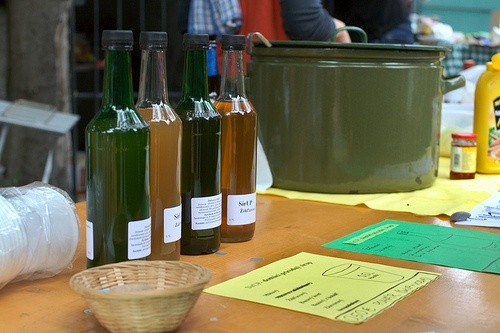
[0,153,500,333]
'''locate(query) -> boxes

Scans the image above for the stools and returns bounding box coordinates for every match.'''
[0,100,82,184]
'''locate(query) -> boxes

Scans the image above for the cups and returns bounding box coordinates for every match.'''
[0,185,80,286]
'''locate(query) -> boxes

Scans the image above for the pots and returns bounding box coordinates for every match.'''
[244,26,465,194]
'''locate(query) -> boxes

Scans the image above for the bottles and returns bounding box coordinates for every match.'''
[176,32,222,256]
[135,30,183,261]
[85,28,151,270]
[214,33,258,242]
[473,54,500,175]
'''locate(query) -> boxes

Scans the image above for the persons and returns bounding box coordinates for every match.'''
[321,0,415,45]
[215,0,351,91]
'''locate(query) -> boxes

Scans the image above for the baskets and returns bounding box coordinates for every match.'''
[69,261,211,333]
